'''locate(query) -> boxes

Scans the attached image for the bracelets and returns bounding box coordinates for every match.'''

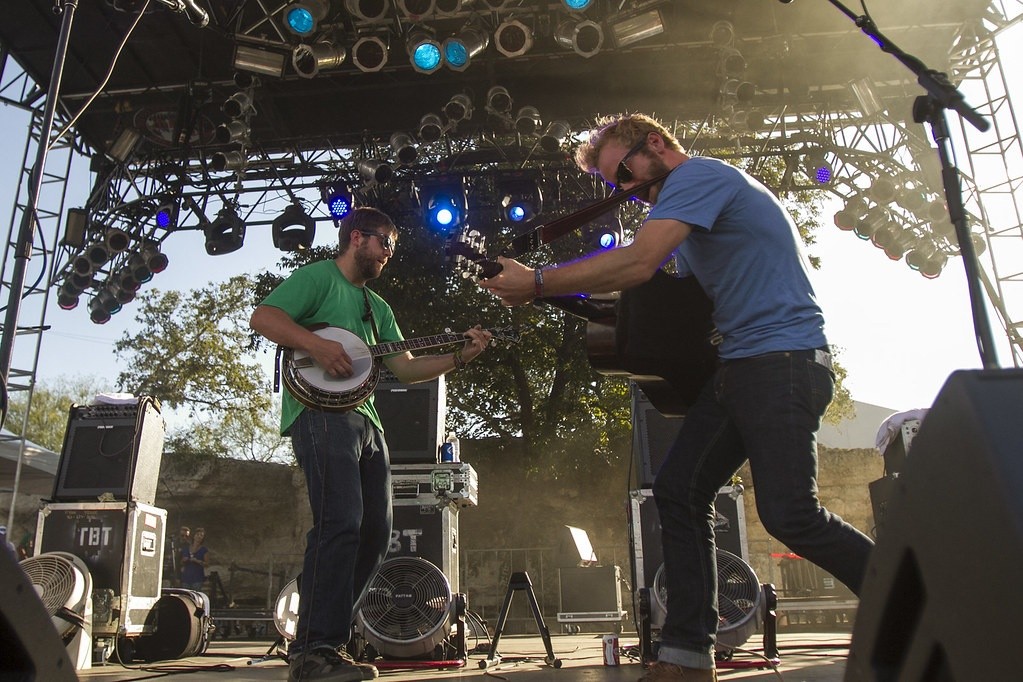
[454,349,466,370]
[533,269,544,301]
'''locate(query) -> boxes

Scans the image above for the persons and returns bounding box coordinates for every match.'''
[477,110,877,681]
[248,208,493,681]
[163,526,192,588]
[178,528,211,592]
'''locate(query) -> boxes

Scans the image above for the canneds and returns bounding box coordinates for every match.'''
[441,443,453,463]
[602,634,620,666]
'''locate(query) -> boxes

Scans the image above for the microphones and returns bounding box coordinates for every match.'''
[180,0,209,28]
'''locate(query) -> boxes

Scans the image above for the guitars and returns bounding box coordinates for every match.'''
[280,322,521,414]
[439,221,718,420]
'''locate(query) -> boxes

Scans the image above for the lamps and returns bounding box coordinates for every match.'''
[833,179,987,280]
[731,111,764,132]
[807,155,835,185]
[719,79,755,103]
[718,48,747,78]
[849,72,887,119]
[196,0,676,261]
[709,20,734,47]
[54,194,188,326]
[101,124,145,171]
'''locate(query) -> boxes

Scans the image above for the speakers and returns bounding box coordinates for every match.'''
[553,524,623,622]
[370,374,447,463]
[52,399,166,505]
[629,381,687,488]
[843,367,1022,682]
[0,541,80,682]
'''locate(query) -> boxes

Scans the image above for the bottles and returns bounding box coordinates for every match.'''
[446,432,460,462]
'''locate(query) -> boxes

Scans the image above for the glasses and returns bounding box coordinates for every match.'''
[617,138,645,183]
[362,228,396,253]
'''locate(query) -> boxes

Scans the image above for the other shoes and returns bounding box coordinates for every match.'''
[287,652,363,682]
[314,648,379,679]
[637,661,717,682]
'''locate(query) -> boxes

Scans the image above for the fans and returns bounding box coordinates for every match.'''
[352,554,470,660]
[636,546,786,672]
[18,554,98,673]
